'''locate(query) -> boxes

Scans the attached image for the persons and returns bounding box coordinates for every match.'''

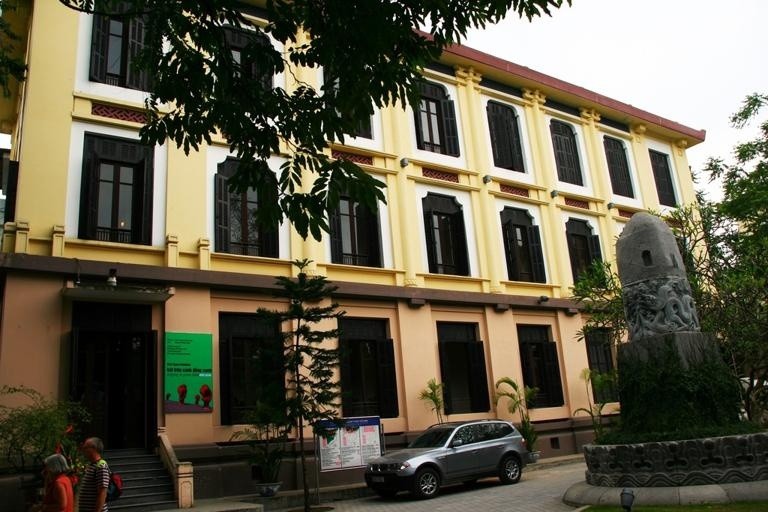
[40,452,76,511]
[77,436,112,511]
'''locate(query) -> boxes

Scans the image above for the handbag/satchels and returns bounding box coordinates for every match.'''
[106,472,123,503]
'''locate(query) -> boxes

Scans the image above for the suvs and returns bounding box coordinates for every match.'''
[363,417,529,499]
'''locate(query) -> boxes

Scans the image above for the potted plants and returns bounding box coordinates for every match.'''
[492,376,543,463]
[229,401,295,496]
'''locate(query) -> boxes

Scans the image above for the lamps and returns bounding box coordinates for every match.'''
[107,274,117,287]
